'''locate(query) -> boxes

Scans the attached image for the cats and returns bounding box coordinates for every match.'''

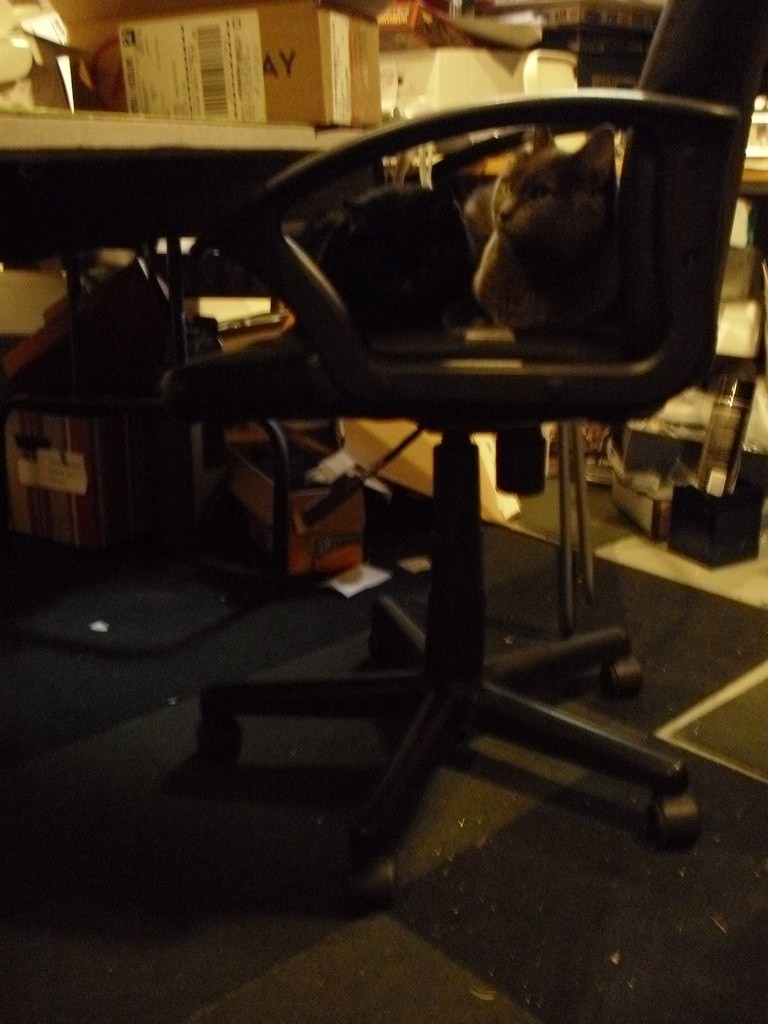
[298,121,621,348]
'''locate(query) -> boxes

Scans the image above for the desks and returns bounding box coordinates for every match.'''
[1,114,378,663]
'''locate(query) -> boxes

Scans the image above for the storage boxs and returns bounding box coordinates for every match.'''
[5,407,141,551]
[223,434,363,579]
[113,2,381,130]
[668,480,765,570]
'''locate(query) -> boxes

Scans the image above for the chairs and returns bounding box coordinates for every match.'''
[154,3,765,910]
